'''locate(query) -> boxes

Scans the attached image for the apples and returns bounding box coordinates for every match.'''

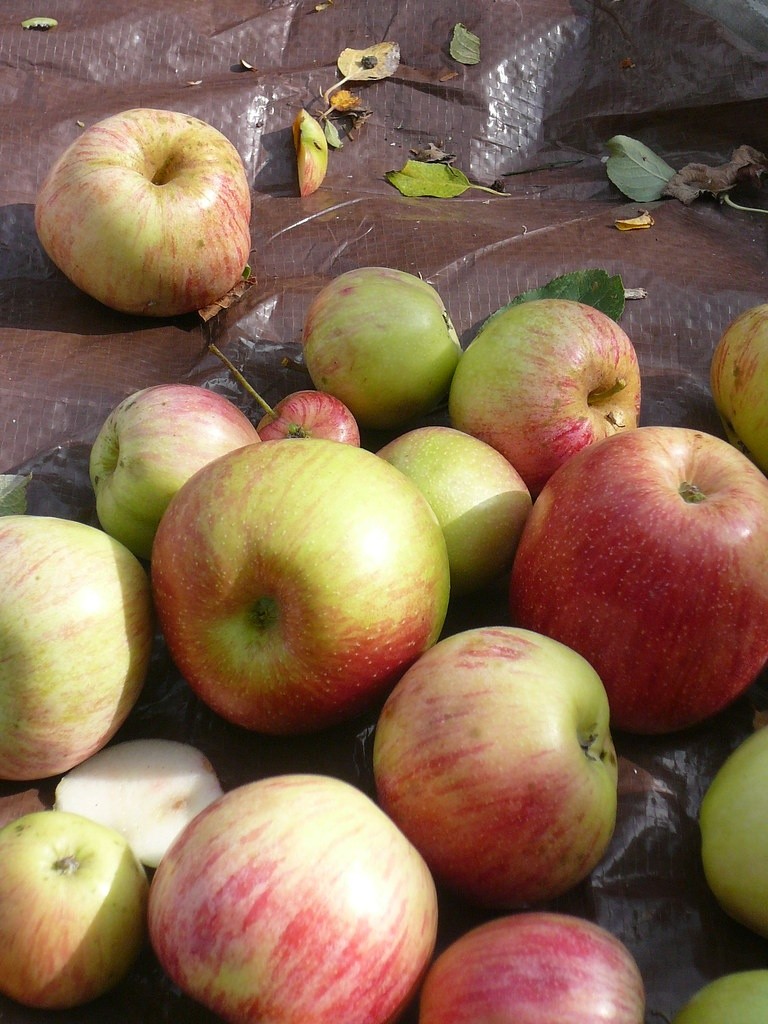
[0,263,768,1024]
[37,108,250,317]
